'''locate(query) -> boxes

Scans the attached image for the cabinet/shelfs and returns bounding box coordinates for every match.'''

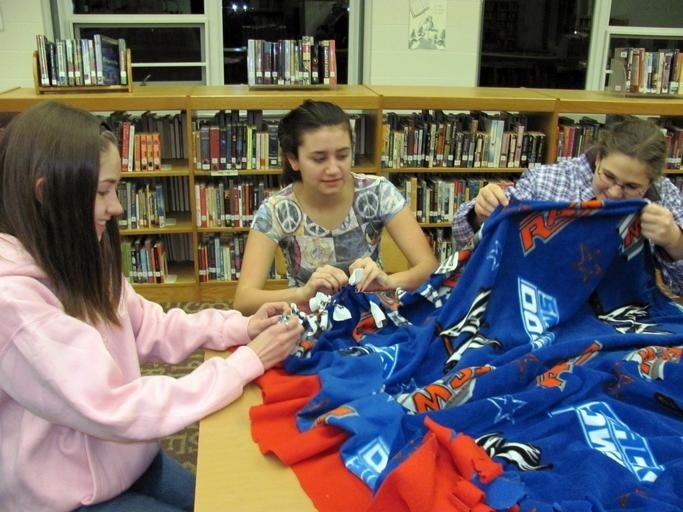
[529,83,683,289]
[1,85,196,307]
[371,83,557,283]
[186,85,381,302]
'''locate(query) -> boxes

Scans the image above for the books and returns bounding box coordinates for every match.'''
[195,173,288,228]
[247,35,339,90]
[34,31,130,87]
[191,109,365,171]
[382,109,546,168]
[612,45,683,95]
[120,234,195,284]
[198,232,287,283]
[117,176,190,229]
[96,110,188,171]
[396,172,520,224]
[557,112,682,191]
[424,228,453,272]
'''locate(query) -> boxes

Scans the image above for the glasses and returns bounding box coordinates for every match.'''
[597,154,653,197]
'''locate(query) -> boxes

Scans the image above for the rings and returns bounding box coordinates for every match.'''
[282,321,289,330]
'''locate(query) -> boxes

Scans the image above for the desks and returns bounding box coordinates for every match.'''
[192,343,683,510]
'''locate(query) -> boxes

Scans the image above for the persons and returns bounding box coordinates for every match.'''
[0,102,306,512]
[319,1,349,50]
[449,117,682,301]
[230,98,442,316]
